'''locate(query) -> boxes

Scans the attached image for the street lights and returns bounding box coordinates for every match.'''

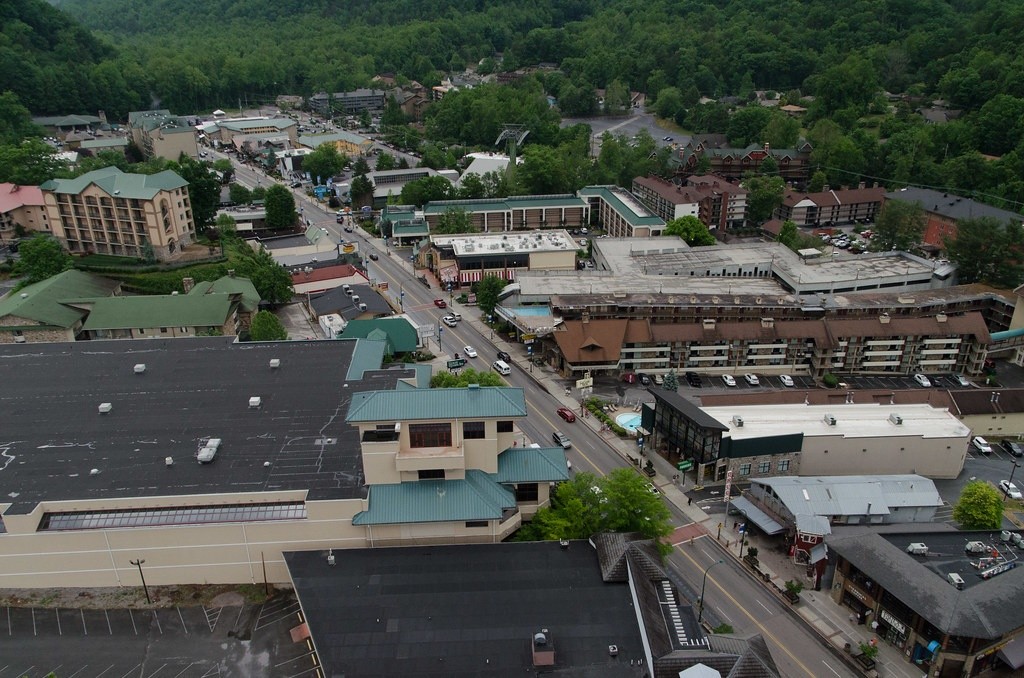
[130,558,166,635]
[700,560,724,623]
[1002,459,1021,502]
[737,506,747,559]
[399,278,413,314]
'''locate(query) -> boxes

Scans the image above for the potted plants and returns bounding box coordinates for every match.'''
[643,459,656,477]
[782,580,805,605]
[856,641,879,671]
[743,546,760,569]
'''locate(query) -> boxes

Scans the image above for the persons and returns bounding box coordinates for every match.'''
[668,472,753,551]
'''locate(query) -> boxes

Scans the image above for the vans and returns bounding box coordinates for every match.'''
[493,360,512,376]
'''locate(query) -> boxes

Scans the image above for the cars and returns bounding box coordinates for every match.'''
[652,373,664,385]
[664,372,672,380]
[644,482,661,499]
[464,345,478,358]
[722,373,736,386]
[552,432,572,451]
[590,486,609,506]
[929,376,945,387]
[685,371,703,386]
[443,315,457,326]
[780,375,794,386]
[337,216,344,223]
[1002,439,1022,458]
[556,409,576,423]
[914,373,931,388]
[638,373,650,384]
[450,311,462,321]
[744,373,759,385]
[434,299,446,308]
[973,436,992,455]
[952,373,969,386]
[565,458,572,470]
[370,252,378,260]
[497,351,510,363]
[999,479,1023,502]
[623,373,637,383]
[344,226,353,233]
